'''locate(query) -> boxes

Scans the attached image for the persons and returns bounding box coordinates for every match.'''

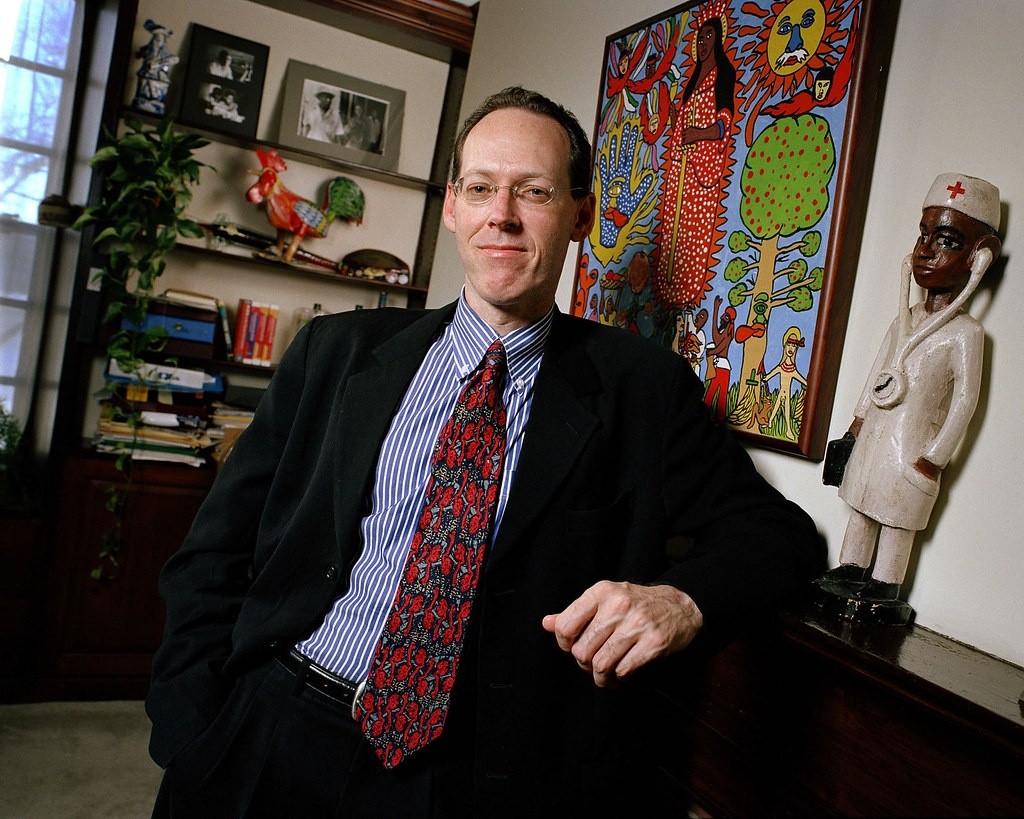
[143,86,819,819]
[821,172,1000,600]
[200,87,239,120]
[303,88,381,151]
[135,19,180,101]
[208,54,233,79]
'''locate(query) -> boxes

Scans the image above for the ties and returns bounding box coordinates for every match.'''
[354,338,509,772]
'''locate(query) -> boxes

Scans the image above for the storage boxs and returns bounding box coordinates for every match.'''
[104,303,224,393]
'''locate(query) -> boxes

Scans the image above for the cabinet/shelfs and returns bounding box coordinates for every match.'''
[37,0,480,702]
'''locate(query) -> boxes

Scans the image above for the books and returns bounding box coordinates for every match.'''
[161,289,279,367]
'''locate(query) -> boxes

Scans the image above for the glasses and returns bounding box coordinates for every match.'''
[448,173,584,207]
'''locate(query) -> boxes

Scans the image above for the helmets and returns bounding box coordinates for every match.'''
[313,85,336,98]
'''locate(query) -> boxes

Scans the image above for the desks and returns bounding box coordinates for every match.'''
[638,623,1024,819]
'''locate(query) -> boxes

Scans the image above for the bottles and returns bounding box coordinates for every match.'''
[38,194,71,228]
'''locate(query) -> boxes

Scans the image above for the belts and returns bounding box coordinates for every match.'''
[273,649,358,711]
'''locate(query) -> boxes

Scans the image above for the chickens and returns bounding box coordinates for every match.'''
[245,146,366,265]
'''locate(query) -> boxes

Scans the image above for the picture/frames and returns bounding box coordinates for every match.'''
[173,21,268,138]
[278,58,407,171]
[568,0,906,464]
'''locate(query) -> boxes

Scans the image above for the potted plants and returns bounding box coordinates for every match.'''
[0,435,48,706]
